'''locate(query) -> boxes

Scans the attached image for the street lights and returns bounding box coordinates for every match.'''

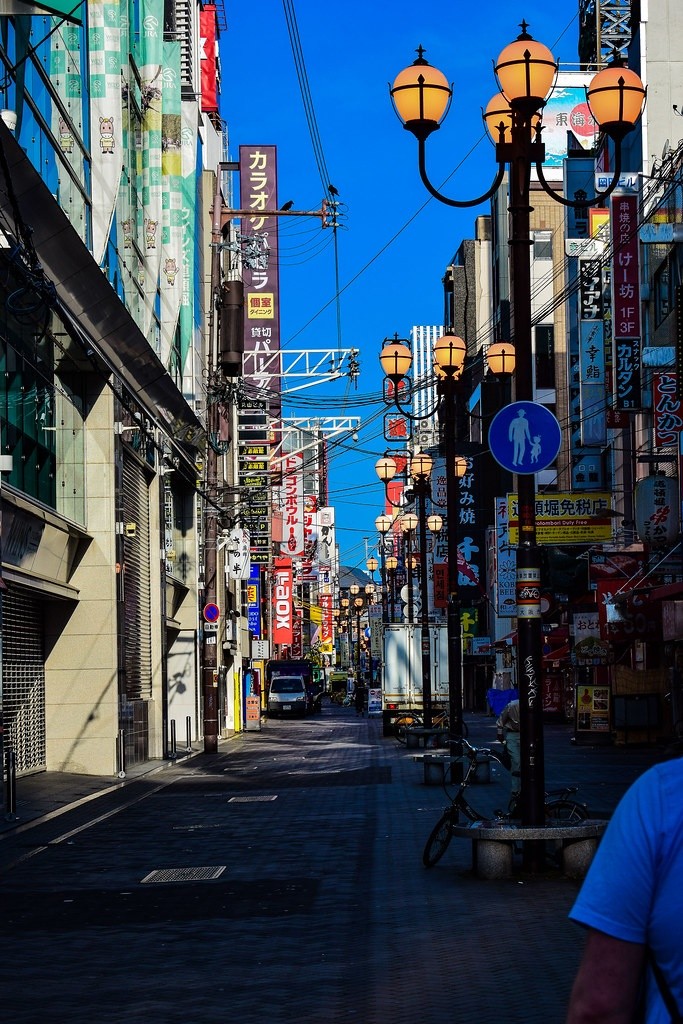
[387,17,649,883]
[340,326,517,786]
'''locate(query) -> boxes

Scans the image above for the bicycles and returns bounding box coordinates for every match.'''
[391,702,469,745]
[422,736,593,868]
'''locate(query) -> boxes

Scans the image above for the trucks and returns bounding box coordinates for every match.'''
[381,623,463,737]
[329,672,348,704]
[265,659,326,720]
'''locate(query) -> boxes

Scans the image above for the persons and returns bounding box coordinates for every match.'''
[353,681,366,718]
[565,749,683,1024]
[496,698,522,799]
